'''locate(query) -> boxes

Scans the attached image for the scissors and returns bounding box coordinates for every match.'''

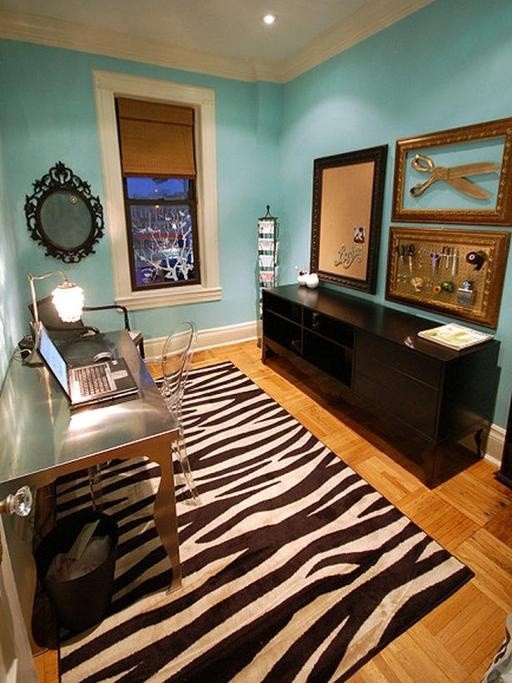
[410,155,500,200]
[396,244,415,274]
[430,252,441,275]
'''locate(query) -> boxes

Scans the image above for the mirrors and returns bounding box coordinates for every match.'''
[21,158,106,265]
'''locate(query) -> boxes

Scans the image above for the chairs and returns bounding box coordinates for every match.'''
[23,296,199,511]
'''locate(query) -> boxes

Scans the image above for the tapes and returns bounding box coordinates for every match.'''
[466,252,484,271]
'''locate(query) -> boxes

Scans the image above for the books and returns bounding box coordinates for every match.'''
[416,321,495,351]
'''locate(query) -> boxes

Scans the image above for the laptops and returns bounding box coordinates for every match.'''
[31,322,139,411]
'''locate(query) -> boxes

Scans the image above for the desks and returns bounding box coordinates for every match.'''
[0,328,188,657]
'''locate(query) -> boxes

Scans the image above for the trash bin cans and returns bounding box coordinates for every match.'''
[35,508,120,633]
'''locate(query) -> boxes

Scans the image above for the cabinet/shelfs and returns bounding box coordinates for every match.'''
[260,280,501,490]
[255,212,280,322]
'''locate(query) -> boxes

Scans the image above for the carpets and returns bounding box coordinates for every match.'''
[52,358,478,682]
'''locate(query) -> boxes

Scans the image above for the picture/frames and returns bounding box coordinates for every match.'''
[385,226,511,331]
[308,143,389,295]
[390,118,510,227]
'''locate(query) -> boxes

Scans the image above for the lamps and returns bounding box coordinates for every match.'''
[17,269,89,367]
